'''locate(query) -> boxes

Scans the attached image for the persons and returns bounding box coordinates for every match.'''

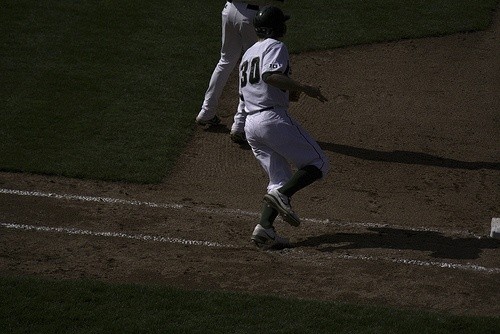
[238,7,330,246]
[195,0,264,143]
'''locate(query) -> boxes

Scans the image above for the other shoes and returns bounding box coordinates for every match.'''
[230,130,247,141]
[195,115,227,126]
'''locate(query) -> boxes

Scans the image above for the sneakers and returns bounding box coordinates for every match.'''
[263,189,301,227]
[250,224,290,248]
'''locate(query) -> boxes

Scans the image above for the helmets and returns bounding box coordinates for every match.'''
[254,6,291,27]
